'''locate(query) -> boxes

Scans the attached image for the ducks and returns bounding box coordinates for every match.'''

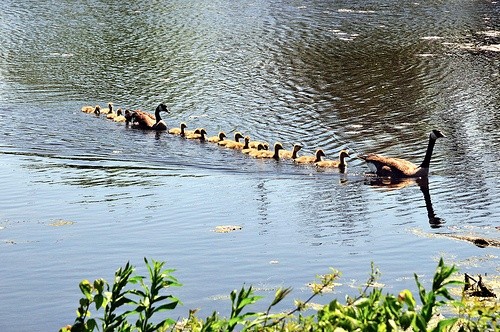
[242,143,265,155]
[226,136,249,149]
[188,129,207,140]
[100,103,114,114]
[184,128,201,136]
[359,128,447,177]
[252,142,285,160]
[128,103,170,129]
[294,150,326,162]
[315,150,351,169]
[278,145,304,160]
[206,132,228,142]
[218,132,245,144]
[169,123,188,134]
[115,110,130,122]
[107,108,122,119]
[250,141,269,150]
[81,105,100,113]
